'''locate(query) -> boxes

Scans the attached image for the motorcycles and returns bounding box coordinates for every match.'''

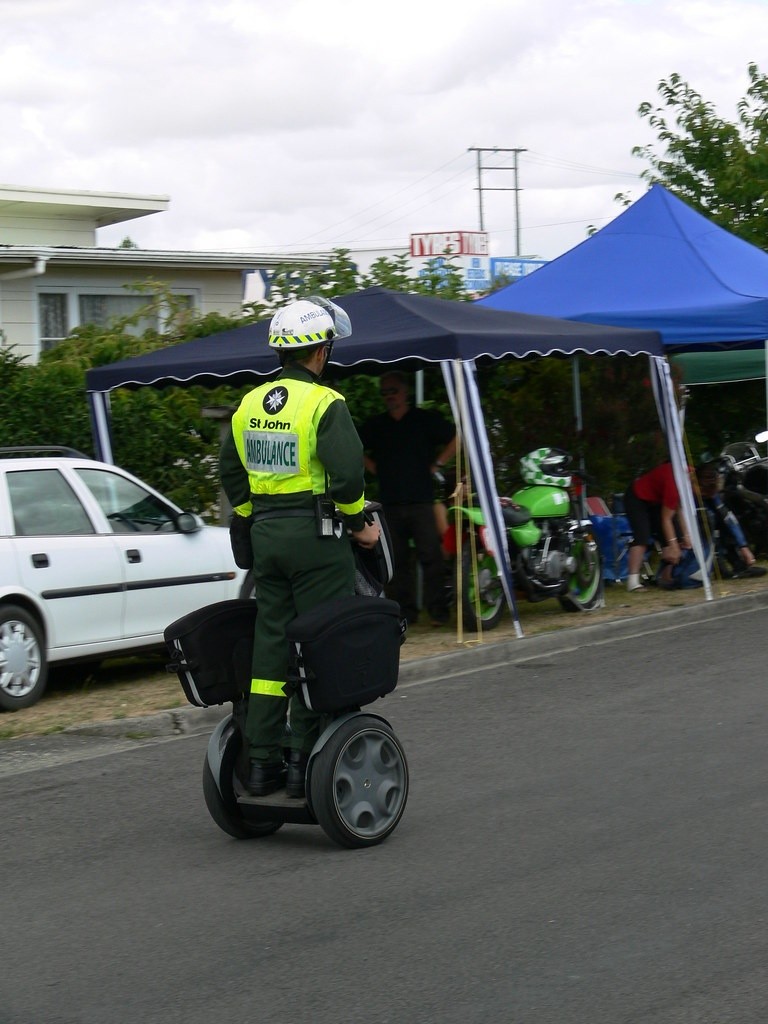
[701,431,768,554]
[444,446,604,632]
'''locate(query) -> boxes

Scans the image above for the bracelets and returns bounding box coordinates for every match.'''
[664,537,681,543]
[433,459,445,469]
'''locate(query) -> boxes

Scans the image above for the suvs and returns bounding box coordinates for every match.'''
[0,441,255,714]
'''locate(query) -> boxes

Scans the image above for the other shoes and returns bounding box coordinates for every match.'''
[431,619,446,626]
[677,576,705,590]
[284,766,305,801]
[248,764,285,797]
[731,562,768,578]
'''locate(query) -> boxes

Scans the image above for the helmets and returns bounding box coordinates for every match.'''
[267,297,353,351]
[516,446,574,489]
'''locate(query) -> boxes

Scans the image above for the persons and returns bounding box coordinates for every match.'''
[366,374,456,626]
[622,457,723,593]
[218,295,381,798]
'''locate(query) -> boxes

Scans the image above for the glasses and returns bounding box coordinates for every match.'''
[378,386,401,398]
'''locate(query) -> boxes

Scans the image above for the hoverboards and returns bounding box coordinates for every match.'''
[161,498,411,851]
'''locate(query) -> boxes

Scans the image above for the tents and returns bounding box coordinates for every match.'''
[81,183,768,644]
